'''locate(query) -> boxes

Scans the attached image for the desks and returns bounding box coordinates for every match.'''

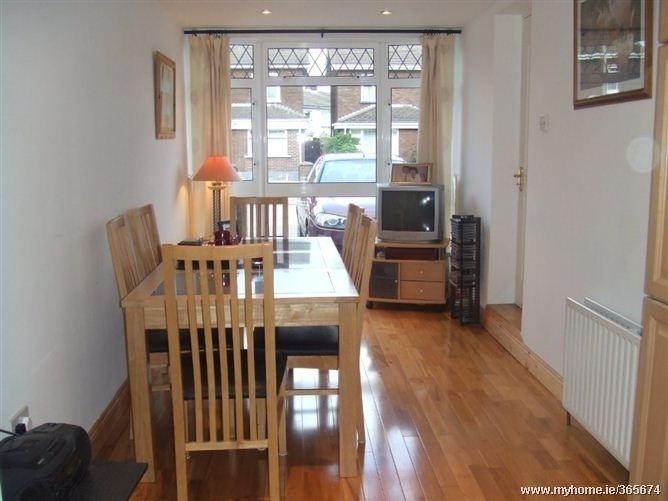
[121,234,359,486]
[64,460,149,501]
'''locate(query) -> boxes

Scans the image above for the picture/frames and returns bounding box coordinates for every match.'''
[389,162,433,187]
[572,0,653,110]
[155,50,176,140]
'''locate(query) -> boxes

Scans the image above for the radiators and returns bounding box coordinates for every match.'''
[561,296,642,486]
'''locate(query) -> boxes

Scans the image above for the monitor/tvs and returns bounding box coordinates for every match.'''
[375,182,444,244]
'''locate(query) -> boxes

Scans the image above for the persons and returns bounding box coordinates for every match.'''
[409,167,425,182]
[396,166,413,181]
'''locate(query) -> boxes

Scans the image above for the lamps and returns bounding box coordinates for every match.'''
[193,153,241,241]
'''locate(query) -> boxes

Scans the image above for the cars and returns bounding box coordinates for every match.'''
[295,152,408,247]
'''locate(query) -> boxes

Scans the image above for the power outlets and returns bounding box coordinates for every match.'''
[10,405,33,437]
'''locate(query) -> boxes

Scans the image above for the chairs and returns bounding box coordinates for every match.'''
[104,214,241,442]
[228,193,288,238]
[339,203,365,270]
[127,202,166,284]
[248,213,376,455]
[160,245,290,501]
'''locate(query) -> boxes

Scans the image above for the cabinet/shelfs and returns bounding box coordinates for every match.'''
[366,240,450,311]
[449,214,482,324]
